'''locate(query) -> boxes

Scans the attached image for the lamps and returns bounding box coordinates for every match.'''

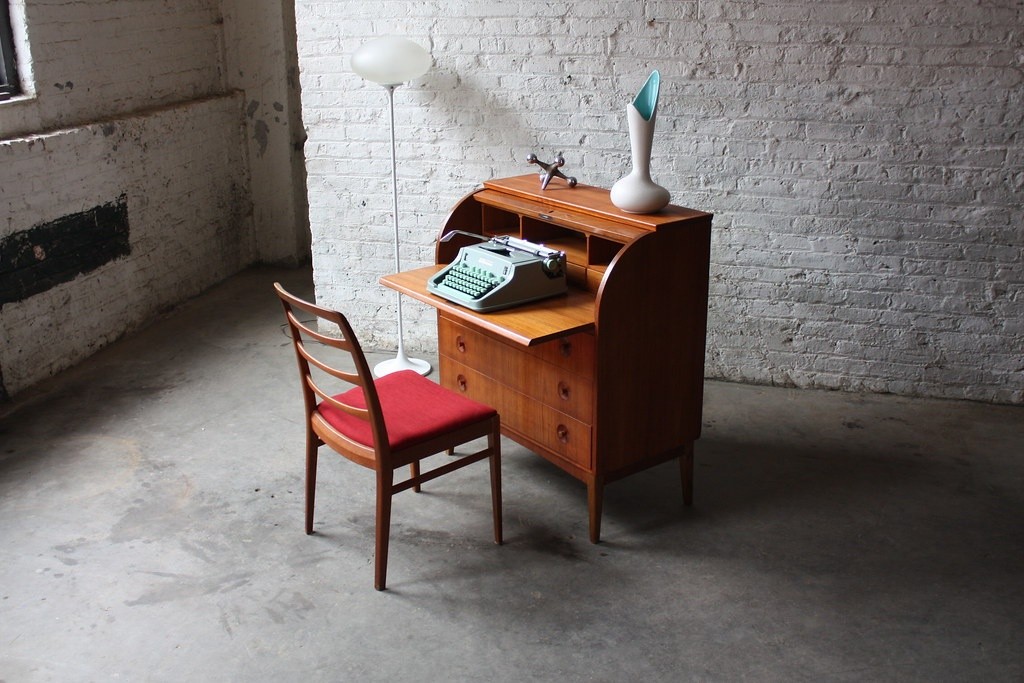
[350,35,432,377]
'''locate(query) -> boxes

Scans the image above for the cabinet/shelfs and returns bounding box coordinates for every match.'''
[380,173,713,544]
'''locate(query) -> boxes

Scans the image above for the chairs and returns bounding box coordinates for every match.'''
[274,282,503,591]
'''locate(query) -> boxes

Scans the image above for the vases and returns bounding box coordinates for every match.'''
[610,69,670,215]
